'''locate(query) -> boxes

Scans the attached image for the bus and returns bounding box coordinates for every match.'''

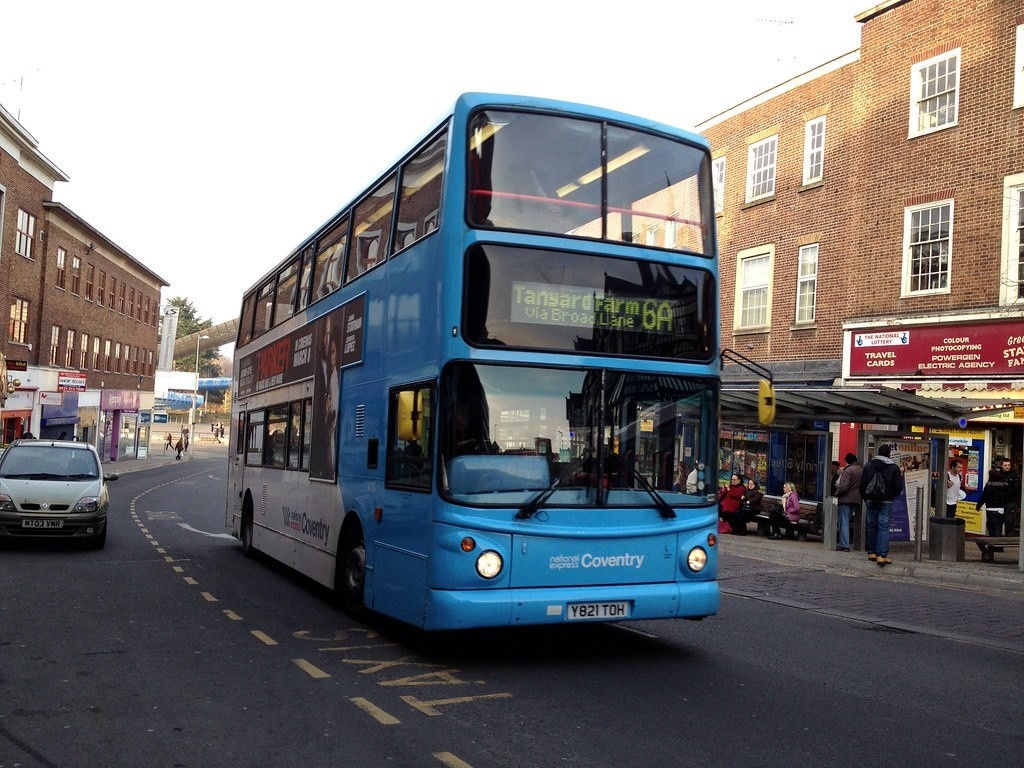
[223,90,777,634]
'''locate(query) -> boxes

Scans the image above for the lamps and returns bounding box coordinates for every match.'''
[86,243,97,255]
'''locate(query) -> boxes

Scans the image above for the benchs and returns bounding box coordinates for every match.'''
[965,537,1020,563]
[755,495,823,542]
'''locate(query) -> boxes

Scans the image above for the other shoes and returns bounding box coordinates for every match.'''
[992,548,1004,552]
[868,553,876,560]
[768,534,783,540]
[836,545,850,552]
[876,556,892,566]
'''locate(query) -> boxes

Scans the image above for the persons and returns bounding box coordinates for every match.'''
[166,433,174,450]
[859,446,902,565]
[175,438,184,460]
[677,461,697,494]
[184,433,189,452]
[832,453,863,553]
[182,427,188,436]
[769,482,800,540]
[976,458,1021,553]
[719,473,762,536]
[947,459,964,518]
[309,315,339,482]
[211,422,224,444]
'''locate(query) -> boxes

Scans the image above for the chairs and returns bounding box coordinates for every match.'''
[272,429,284,446]
[67,458,90,475]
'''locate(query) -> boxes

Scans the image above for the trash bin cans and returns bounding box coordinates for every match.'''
[928,516,966,561]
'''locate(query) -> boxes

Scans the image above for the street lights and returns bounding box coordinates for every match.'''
[190,335,210,457]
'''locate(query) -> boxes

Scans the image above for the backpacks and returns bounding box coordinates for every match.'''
[865,470,886,500]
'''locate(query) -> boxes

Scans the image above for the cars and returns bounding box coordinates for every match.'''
[0,438,120,549]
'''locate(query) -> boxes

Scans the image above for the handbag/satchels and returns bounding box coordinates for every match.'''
[718,520,732,533]
[179,451,183,457]
[768,504,781,518]
